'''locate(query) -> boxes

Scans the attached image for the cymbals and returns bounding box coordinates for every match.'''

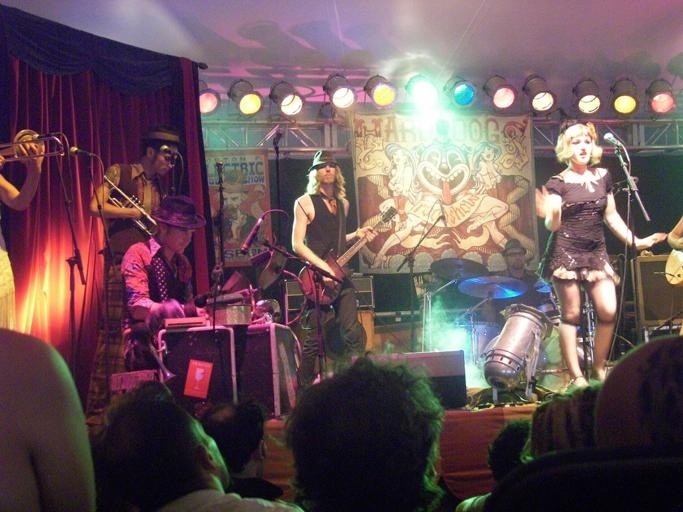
[430,259,488,281]
[457,275,529,298]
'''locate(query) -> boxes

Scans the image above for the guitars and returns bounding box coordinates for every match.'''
[299,207,397,304]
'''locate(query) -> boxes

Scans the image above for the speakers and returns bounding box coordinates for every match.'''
[352,350,467,407]
[632,257,683,327]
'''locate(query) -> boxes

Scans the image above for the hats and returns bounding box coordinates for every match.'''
[307,150,341,172]
[139,126,186,147]
[500,238,527,257]
[149,194,207,230]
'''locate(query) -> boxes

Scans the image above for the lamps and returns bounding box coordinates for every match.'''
[198,72,677,118]
[480,302,554,405]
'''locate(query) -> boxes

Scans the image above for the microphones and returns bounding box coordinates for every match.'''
[604,132,623,147]
[440,204,448,226]
[160,144,177,157]
[70,146,93,158]
[216,160,224,183]
[241,216,263,254]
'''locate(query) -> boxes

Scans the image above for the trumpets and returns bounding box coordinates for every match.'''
[0,132,69,162]
[101,175,158,235]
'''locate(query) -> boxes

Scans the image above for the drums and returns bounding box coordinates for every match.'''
[447,322,500,359]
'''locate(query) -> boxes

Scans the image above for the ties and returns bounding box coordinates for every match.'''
[328,199,337,217]
